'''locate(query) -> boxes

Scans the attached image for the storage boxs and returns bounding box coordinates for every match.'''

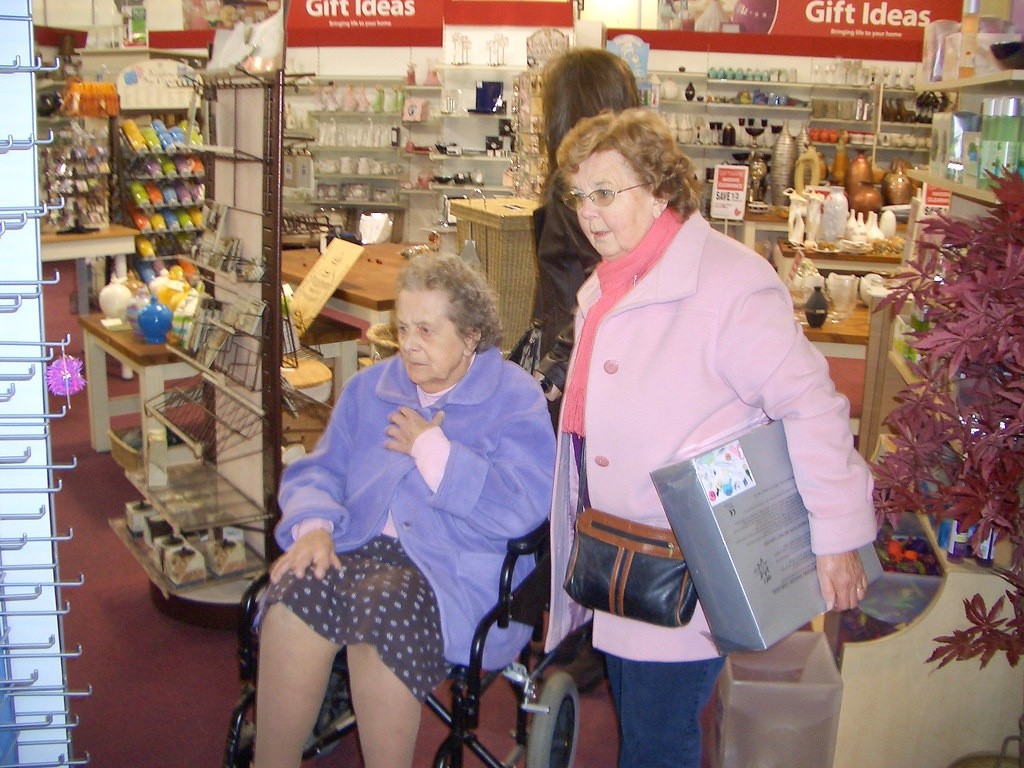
[713,632,844,768]
[650,419,886,654]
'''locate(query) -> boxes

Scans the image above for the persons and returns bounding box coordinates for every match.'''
[254,256,555,767]
[509,50,641,698]
[544,108,878,767]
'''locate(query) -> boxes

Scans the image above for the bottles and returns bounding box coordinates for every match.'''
[122,117,206,285]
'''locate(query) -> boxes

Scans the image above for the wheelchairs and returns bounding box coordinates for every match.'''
[222,321,598,768]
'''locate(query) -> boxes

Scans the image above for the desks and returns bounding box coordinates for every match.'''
[41,223,141,320]
[75,313,203,489]
[280,242,411,311]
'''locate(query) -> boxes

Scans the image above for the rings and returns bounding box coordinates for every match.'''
[857,587,863,592]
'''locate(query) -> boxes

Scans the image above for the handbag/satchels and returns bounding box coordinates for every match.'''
[562,509,699,627]
[506,328,542,378]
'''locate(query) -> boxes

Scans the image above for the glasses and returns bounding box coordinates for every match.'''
[559,182,656,211]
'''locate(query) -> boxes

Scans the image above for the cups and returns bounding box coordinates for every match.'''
[772,126,782,134]
[749,119,754,126]
[709,123,716,129]
[716,123,723,130]
[739,119,744,126]
[762,120,767,126]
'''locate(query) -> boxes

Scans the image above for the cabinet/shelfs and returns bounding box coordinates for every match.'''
[636,70,1024,768]
[0,0,575,768]
